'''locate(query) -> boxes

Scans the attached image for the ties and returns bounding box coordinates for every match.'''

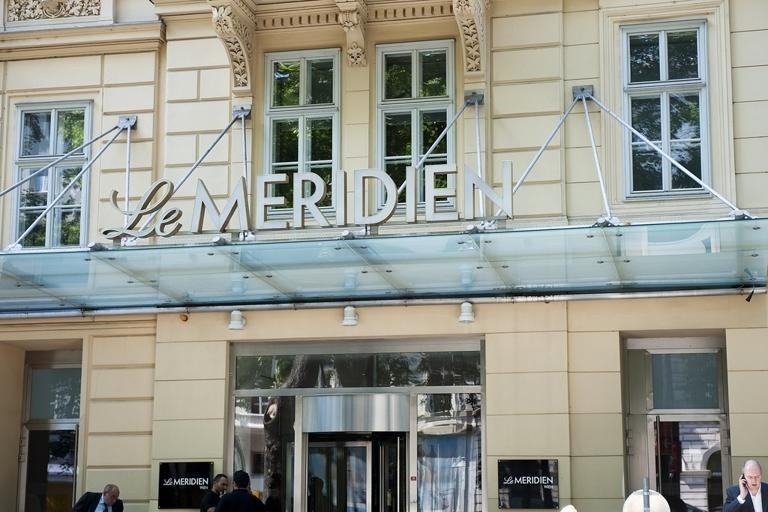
[103,502,109,512]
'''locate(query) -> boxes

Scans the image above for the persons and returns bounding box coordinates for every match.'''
[200,470,266,512]
[71,484,124,512]
[724,459,768,512]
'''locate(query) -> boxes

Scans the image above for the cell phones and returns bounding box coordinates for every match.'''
[740,474,746,488]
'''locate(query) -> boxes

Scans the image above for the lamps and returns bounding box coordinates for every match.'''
[341,306,359,326]
[457,301,475,324]
[227,310,245,331]
